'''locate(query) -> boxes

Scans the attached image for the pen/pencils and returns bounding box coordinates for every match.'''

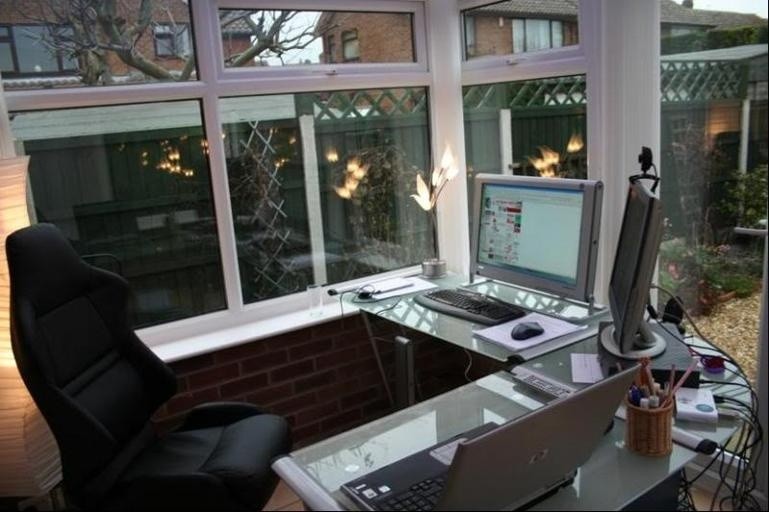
[629,358,699,408]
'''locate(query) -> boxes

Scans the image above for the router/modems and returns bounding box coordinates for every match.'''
[676,385,718,425]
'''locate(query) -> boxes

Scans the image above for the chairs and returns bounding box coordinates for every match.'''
[6,222,295,512]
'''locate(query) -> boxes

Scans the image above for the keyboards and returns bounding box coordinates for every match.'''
[414,287,526,326]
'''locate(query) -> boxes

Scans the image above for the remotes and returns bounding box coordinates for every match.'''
[515,371,569,397]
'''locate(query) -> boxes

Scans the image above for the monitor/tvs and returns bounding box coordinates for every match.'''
[469,172,603,303]
[601,182,667,359]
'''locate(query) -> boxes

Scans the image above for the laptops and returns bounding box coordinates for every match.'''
[339,364,645,511]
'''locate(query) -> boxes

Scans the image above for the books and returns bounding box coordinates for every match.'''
[471,312,588,354]
[670,386,721,425]
[365,276,440,303]
[368,277,413,294]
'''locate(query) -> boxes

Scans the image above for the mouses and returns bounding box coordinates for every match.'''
[511,321,543,340]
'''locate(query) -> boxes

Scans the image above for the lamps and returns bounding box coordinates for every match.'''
[410,146,458,278]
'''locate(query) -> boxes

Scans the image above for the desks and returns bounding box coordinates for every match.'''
[270,280,753,512]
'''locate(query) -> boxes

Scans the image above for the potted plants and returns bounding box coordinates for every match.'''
[698,264,723,316]
